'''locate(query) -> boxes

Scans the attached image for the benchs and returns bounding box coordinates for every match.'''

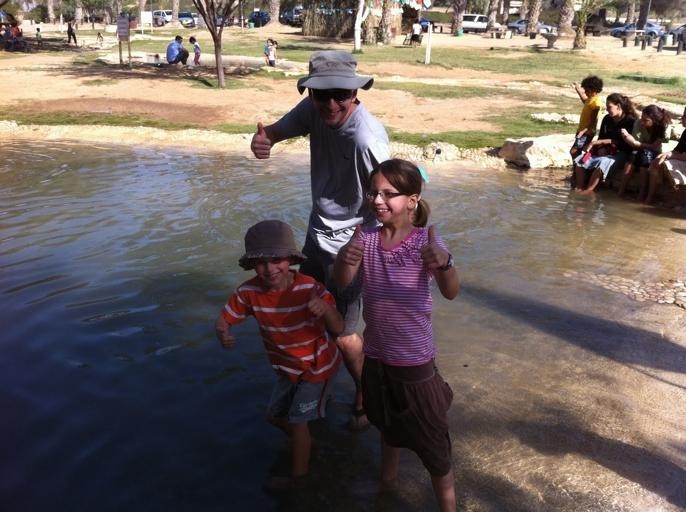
[488,30,512,40]
[434,25,443,32]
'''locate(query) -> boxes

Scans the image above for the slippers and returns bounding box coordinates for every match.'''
[348,404,373,432]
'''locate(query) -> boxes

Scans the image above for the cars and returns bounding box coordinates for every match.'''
[670,21,686,48]
[508,16,553,34]
[419,14,430,32]
[85,3,305,29]
[608,20,666,41]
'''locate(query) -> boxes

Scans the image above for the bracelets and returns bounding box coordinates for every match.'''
[441,252,456,272]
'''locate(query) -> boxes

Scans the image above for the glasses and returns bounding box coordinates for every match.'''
[364,191,406,199]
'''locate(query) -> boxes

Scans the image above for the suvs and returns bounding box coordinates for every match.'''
[460,13,503,33]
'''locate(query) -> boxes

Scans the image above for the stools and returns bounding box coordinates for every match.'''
[621,35,652,50]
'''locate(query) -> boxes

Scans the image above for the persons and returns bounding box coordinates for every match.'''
[0,18,107,58]
[249,50,400,428]
[645,107,686,209]
[576,93,639,194]
[411,21,423,46]
[165,35,189,67]
[269,41,277,67]
[265,39,272,66]
[188,37,201,66]
[329,158,464,512]
[217,219,346,488]
[616,104,673,202]
[570,75,604,186]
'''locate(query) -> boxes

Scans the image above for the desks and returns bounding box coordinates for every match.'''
[491,26,507,30]
[540,32,566,47]
[625,29,645,33]
[429,20,440,32]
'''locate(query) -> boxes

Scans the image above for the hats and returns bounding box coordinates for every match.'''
[238,220,307,271]
[296,49,374,93]
